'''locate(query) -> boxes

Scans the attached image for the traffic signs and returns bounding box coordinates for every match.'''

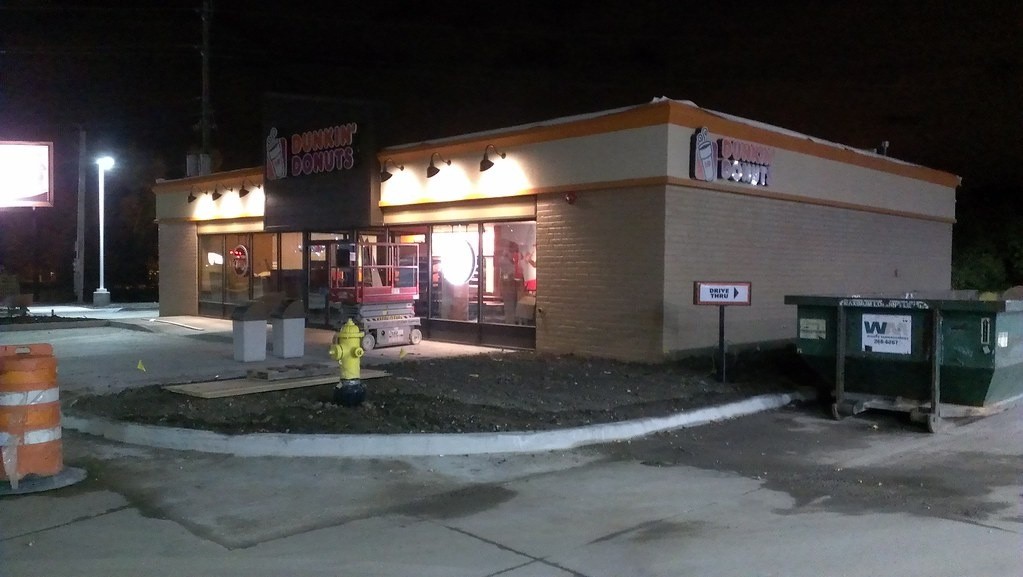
[694,281,752,306]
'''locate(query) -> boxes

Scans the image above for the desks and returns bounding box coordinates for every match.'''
[473,295,504,301]
[469,301,504,308]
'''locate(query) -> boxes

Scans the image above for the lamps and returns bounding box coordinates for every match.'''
[381,158,404,183]
[188,185,208,203]
[427,152,451,178]
[480,144,506,171]
[239,178,261,198]
[212,182,233,200]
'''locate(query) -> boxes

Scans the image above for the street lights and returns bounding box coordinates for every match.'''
[93,153,116,309]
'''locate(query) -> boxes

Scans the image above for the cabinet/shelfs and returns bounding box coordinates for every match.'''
[400,255,486,303]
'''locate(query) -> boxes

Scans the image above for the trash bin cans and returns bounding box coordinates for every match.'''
[270,298,306,359]
[231,300,268,362]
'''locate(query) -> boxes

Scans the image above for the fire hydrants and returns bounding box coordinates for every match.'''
[329,316,368,408]
